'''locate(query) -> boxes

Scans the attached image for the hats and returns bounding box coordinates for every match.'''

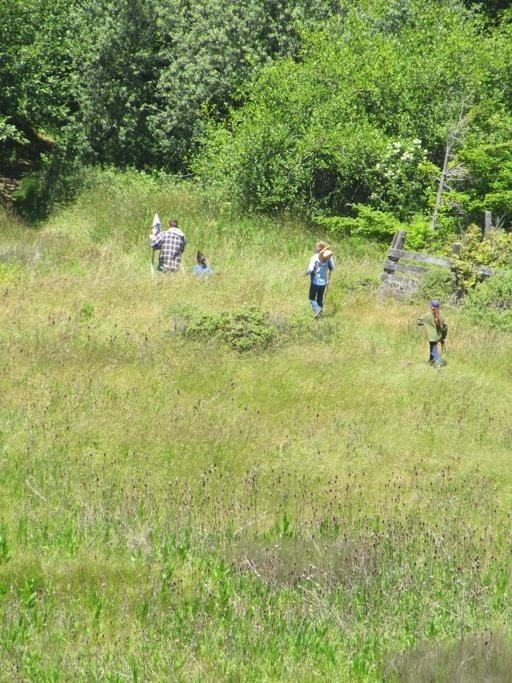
[431,299,440,307]
[318,245,333,263]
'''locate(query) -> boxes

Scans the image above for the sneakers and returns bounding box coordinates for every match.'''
[313,307,322,318]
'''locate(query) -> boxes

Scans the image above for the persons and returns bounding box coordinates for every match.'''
[306,241,336,320]
[149,219,212,274]
[417,300,448,368]
